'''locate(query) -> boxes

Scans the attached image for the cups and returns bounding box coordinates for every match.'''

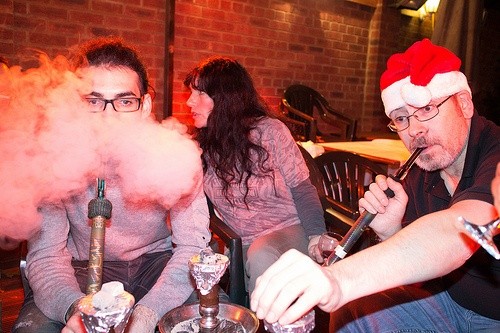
[318,232,343,266]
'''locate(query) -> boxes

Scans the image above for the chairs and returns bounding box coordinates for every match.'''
[18,84,388,309]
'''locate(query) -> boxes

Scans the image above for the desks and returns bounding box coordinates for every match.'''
[311,139,412,186]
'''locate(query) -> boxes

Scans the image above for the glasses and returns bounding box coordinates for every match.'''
[81,97,143,114]
[386,93,453,133]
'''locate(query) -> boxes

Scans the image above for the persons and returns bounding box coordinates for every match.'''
[250,39,500,333]
[183,55,340,291]
[12,40,212,333]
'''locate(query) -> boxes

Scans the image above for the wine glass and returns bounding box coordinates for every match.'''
[458,206,500,260]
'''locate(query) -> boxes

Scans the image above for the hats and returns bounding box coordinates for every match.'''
[379,39,473,119]
